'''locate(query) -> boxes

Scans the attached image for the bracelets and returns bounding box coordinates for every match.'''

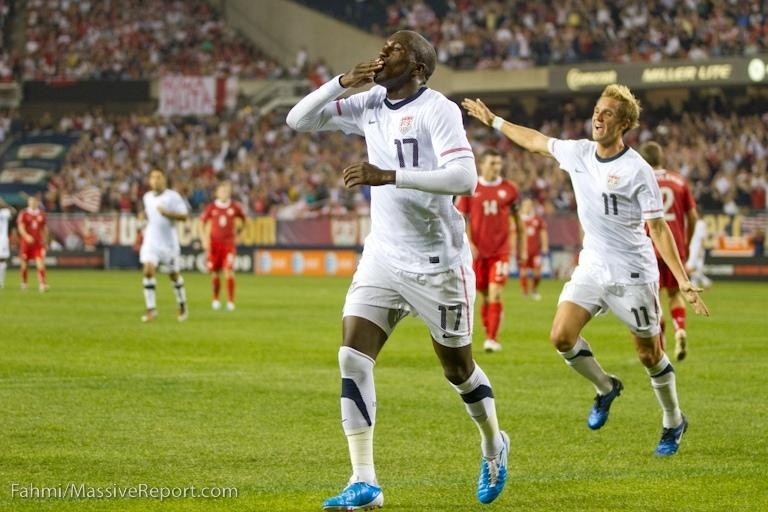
[490,116,504,130]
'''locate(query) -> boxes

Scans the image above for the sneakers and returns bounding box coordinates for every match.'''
[22,283,235,321]
[484,338,500,351]
[674,328,687,361]
[588,375,624,430]
[478,431,510,504]
[655,411,688,456]
[320,476,384,511]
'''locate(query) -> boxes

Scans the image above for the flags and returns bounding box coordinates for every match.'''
[741,210,767,232]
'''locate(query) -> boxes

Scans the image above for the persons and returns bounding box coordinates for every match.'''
[635,141,698,362]
[196,178,246,310]
[514,198,548,301]
[15,195,50,292]
[139,168,189,323]
[451,147,523,351]
[0,0,767,211]
[285,31,510,510]
[0,198,15,288]
[461,83,710,456]
